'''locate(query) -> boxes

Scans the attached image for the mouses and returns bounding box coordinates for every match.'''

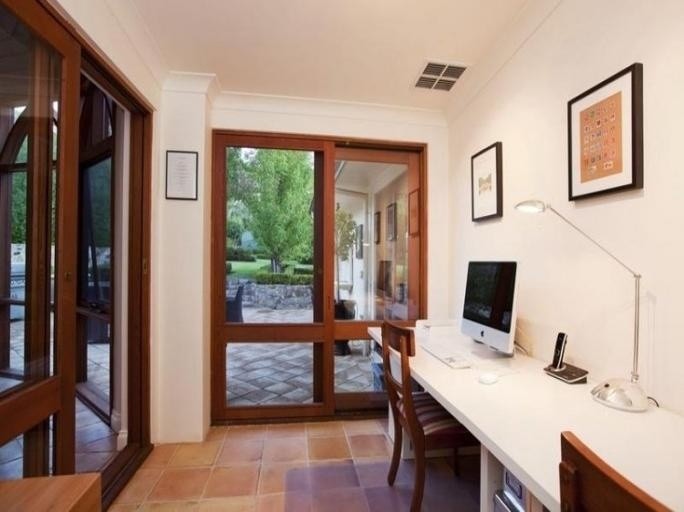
[478,373,499,385]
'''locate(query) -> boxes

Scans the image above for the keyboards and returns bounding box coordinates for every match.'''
[420,339,472,369]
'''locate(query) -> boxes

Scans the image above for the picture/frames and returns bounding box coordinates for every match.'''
[355,225,363,259]
[408,188,419,236]
[566,62,643,201]
[374,210,381,244]
[385,203,396,241]
[471,142,502,222]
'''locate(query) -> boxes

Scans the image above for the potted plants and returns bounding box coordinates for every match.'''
[335,210,361,356]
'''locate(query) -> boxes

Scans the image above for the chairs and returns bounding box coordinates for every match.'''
[559,430,673,512]
[226,284,243,322]
[381,321,481,512]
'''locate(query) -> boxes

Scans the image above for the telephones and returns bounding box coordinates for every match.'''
[544,332,589,384]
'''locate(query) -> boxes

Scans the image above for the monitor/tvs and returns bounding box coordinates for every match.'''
[460,259,519,360]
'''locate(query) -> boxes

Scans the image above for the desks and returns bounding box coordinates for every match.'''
[367,325,684,512]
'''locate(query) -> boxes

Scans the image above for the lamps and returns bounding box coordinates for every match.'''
[515,201,650,411]
